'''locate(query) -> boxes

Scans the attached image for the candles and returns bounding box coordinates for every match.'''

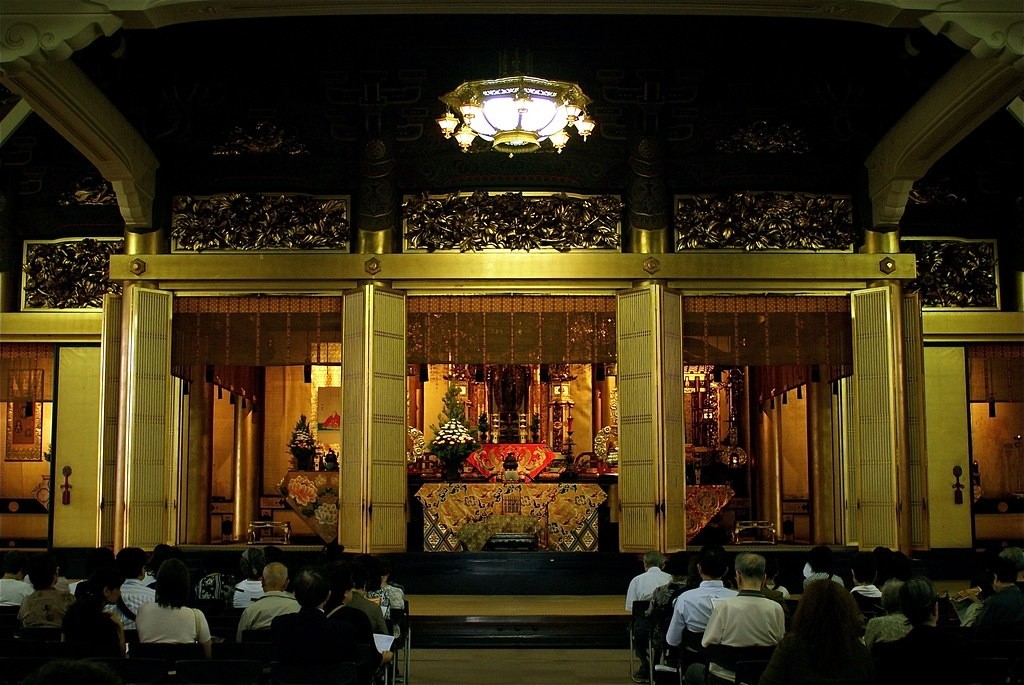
[567,415,574,431]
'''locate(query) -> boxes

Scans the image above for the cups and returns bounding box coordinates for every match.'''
[766,578,775,590]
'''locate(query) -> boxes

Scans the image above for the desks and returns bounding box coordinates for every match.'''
[283,471,339,545]
[686,485,736,546]
[414,483,608,552]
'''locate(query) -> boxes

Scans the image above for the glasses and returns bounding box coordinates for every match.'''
[55,565,61,574]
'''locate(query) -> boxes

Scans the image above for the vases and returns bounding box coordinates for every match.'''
[297,457,313,470]
[440,463,463,482]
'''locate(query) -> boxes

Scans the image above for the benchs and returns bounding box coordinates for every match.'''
[730,520,775,544]
[248,521,290,544]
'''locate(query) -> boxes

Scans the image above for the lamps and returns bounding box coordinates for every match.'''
[433,47,597,158]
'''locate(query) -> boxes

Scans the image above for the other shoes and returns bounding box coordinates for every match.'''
[634,663,650,680]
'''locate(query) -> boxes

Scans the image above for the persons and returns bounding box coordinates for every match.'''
[0,544,405,685]
[624,545,1024,685]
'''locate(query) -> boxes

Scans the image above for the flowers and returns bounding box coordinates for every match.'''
[286,412,317,457]
[428,382,481,462]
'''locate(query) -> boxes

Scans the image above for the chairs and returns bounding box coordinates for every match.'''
[631,592,1024,685]
[1,552,412,685]
